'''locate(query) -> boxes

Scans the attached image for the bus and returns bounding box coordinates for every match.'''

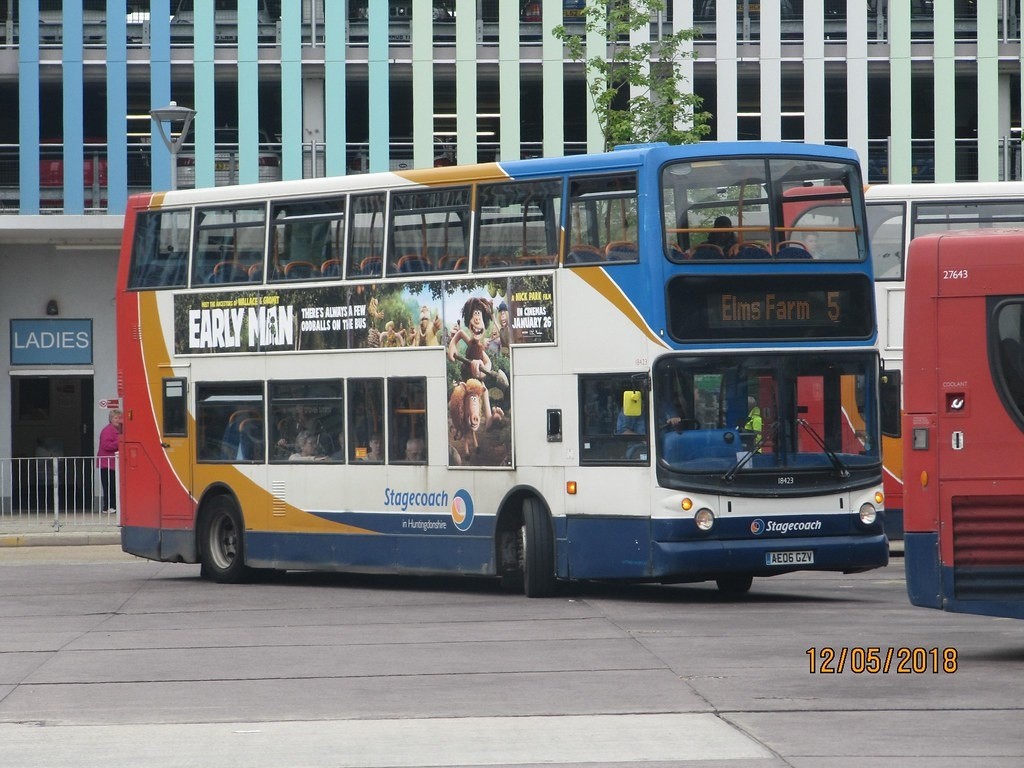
[755,180,1024,620]
[117,142,890,598]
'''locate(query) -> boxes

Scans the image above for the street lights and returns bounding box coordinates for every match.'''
[148,101,198,252]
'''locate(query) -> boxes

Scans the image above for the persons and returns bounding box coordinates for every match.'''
[707,216,739,250]
[803,231,822,260]
[277,382,763,466]
[97,409,120,513]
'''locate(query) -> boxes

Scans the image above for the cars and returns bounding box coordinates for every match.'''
[38,136,106,206]
[349,0,451,48]
[171,0,275,42]
[38,5,134,43]
[520,0,586,47]
[348,137,453,175]
[176,123,280,188]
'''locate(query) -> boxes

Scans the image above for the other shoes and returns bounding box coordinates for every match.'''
[106,508,115,514]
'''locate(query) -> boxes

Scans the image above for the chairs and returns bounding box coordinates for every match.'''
[207,240,812,284]
[220,409,377,460]
